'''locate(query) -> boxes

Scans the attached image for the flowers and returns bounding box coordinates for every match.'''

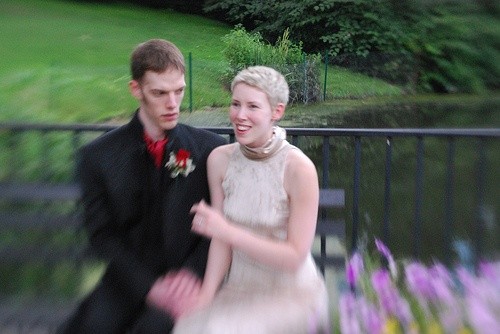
[340,237,500,334]
[165,149,196,178]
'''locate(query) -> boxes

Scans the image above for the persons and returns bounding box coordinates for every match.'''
[173,65,330,334]
[52,39,230,334]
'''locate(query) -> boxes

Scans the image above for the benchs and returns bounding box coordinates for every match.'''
[0,186,345,334]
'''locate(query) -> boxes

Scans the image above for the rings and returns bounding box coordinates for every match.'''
[197,217,204,226]
